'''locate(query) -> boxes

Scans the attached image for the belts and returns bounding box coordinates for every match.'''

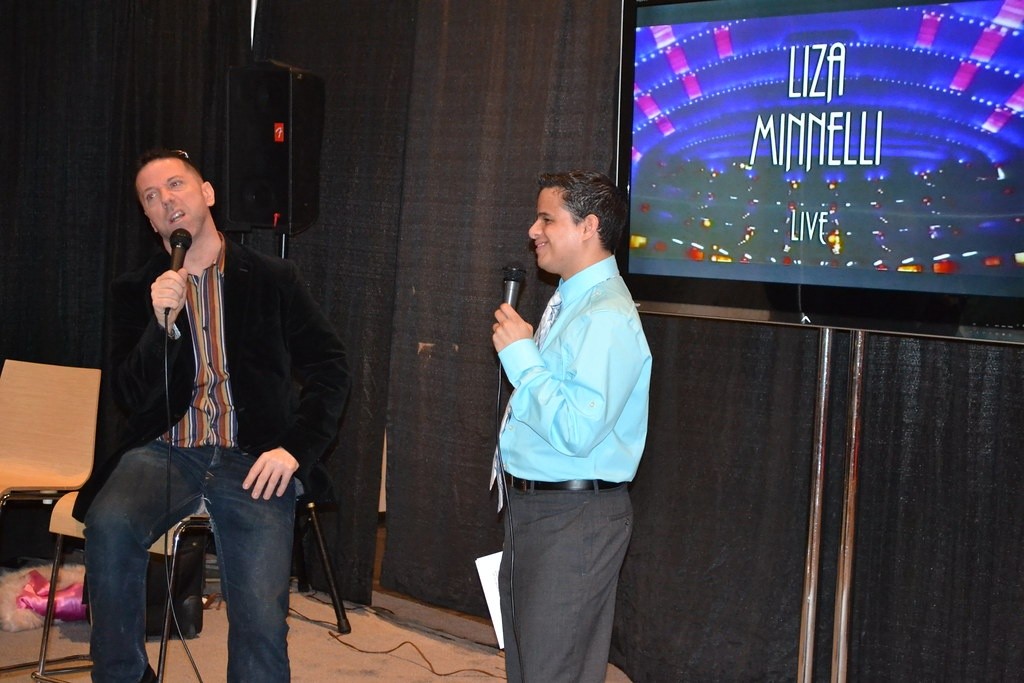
[502,470,625,492]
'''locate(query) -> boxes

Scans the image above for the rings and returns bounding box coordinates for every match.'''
[495,326,499,332]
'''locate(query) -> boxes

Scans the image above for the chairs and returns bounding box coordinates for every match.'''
[1,358,102,674]
[32,488,212,683]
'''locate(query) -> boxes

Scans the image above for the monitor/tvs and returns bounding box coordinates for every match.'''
[615,0,1024,346]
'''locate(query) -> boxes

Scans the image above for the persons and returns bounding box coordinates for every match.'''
[489,171,653,682]
[71,149,354,683]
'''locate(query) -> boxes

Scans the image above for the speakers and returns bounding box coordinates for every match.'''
[145,533,210,636]
[220,60,325,238]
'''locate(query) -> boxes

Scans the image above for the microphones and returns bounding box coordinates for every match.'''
[503,261,526,310]
[163,228,191,315]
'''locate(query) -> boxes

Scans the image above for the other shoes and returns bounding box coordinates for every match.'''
[140,664,158,683]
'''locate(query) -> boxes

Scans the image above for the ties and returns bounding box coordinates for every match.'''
[490,292,562,491]
